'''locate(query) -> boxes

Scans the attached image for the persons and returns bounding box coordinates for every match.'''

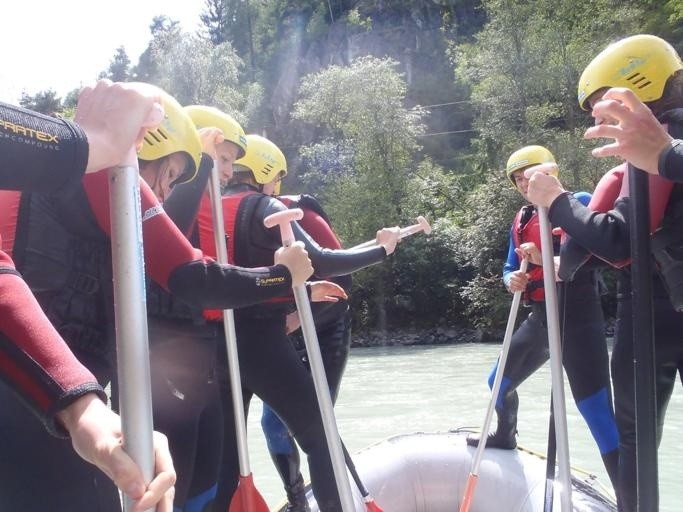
[112,104,248,511]
[262,282,348,303]
[0,80,178,512]
[465,144,617,511]
[555,162,683,511]
[526,34,681,316]
[0,78,316,512]
[584,87,682,181]
[214,135,402,512]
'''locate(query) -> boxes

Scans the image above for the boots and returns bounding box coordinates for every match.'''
[465,406,519,451]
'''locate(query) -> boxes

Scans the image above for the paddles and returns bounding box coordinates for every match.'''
[352,216,432,249]
[544,283,565,511]
[629,163,659,511]
[105,102,155,510]
[209,160,269,512]
[460,259,528,512]
[538,207,571,512]
[339,437,384,511]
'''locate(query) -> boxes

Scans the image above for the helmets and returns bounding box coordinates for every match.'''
[506,144,559,193]
[232,134,288,185]
[124,81,202,185]
[577,34,683,111]
[183,105,248,160]
[272,180,282,196]
